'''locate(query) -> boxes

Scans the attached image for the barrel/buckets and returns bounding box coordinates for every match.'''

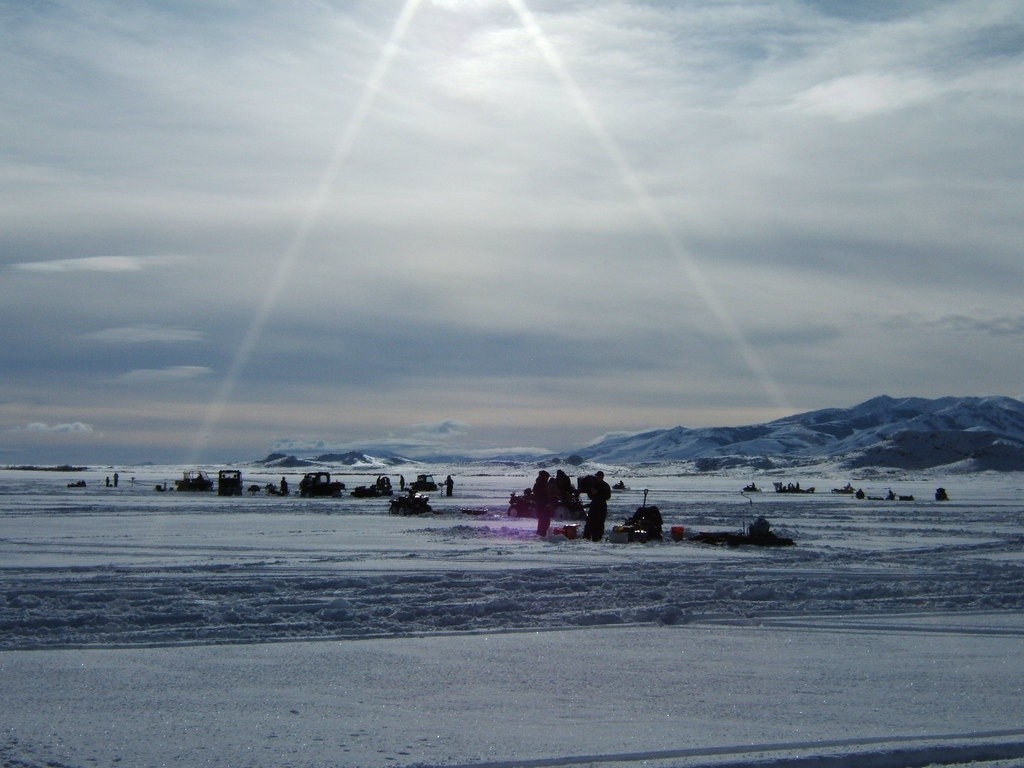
[671,526,684,541]
[553,527,567,536]
[565,524,577,539]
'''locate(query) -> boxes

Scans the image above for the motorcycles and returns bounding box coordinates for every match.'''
[176,470,441,520]
[504,488,584,521]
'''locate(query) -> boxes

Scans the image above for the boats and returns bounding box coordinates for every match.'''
[742,477,951,503]
[725,530,796,549]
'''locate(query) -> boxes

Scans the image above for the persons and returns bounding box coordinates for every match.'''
[618,480,625,487]
[279,476,290,494]
[749,481,756,489]
[582,470,612,544]
[196,473,204,481]
[221,474,226,479]
[550,469,574,506]
[795,482,800,490]
[531,470,553,539]
[231,474,237,479]
[885,489,895,500]
[399,475,406,491]
[105,476,111,486]
[113,472,119,488]
[788,482,791,490]
[779,482,783,490]
[843,482,851,490]
[376,475,382,494]
[440,474,455,497]
